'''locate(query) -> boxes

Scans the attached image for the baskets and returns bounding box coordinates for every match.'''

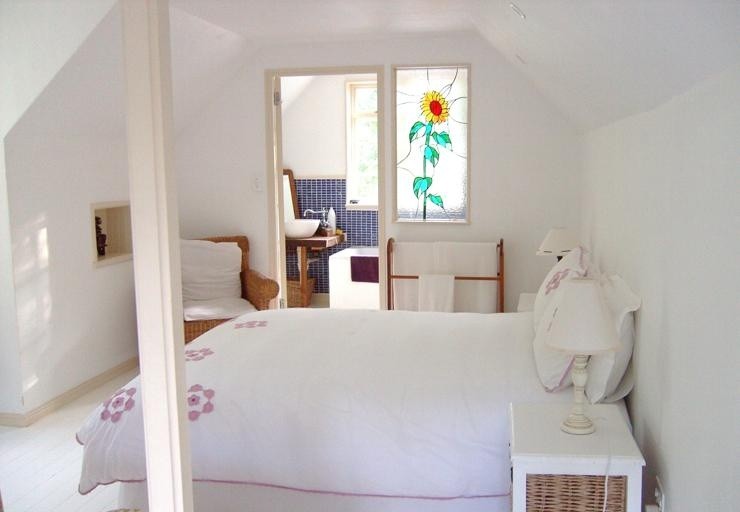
[527,476,627,512]
[287,276,315,307]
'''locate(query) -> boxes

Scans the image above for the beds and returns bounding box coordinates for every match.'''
[118,309,634,512]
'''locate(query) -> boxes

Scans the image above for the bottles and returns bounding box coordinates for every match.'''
[328,206,337,234]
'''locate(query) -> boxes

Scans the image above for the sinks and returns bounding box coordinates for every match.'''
[284,216,320,239]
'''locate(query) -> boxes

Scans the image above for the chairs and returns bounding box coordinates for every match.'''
[185,236,278,343]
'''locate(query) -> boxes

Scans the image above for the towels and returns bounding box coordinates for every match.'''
[392,241,498,314]
[419,275,454,312]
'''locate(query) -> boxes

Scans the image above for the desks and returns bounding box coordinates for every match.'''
[286,234,344,308]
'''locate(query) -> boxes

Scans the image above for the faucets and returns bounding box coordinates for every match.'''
[302,206,329,228]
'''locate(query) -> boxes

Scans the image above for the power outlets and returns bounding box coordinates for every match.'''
[655,476,665,511]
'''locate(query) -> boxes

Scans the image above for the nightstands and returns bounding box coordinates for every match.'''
[510,402,646,512]
[518,293,536,312]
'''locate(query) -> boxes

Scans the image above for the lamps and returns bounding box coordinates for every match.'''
[537,228,578,261]
[545,279,620,434]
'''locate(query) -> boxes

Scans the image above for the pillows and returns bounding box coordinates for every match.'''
[182,241,242,300]
[584,275,641,402]
[532,247,595,390]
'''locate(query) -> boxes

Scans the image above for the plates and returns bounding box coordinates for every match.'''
[349,199,359,205]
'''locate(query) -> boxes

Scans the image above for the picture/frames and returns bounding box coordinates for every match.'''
[389,63,473,226]
